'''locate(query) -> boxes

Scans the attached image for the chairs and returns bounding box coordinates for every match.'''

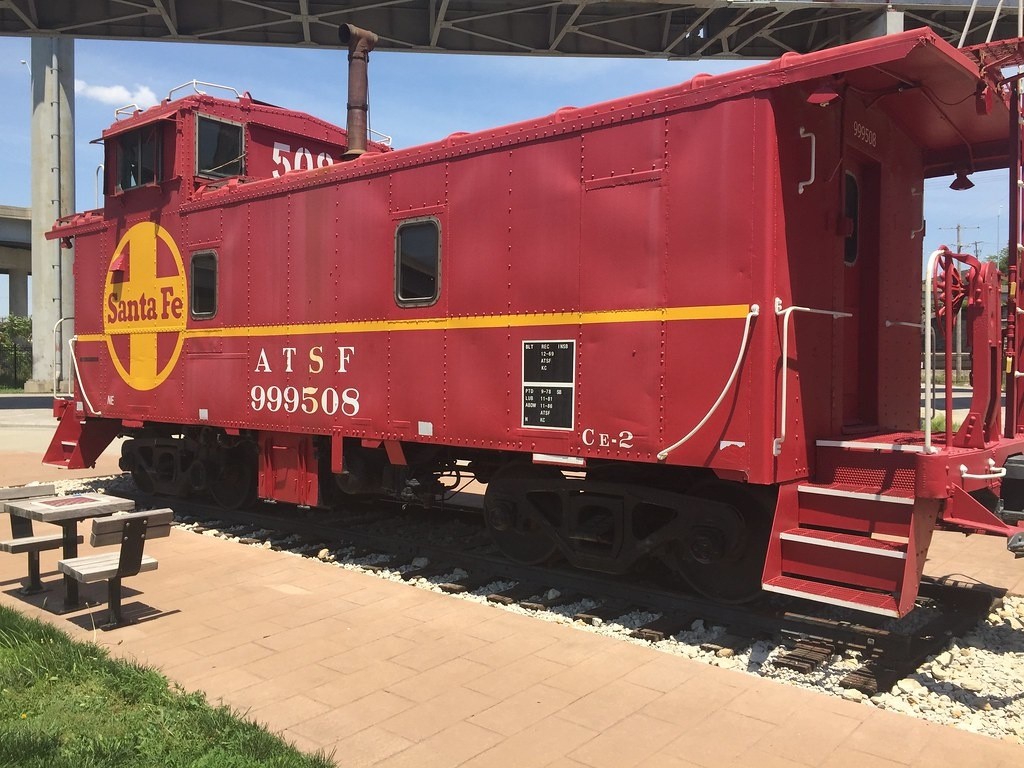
[0,484,83,596]
[57,508,174,632]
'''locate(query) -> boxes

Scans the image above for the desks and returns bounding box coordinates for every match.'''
[4,492,135,611]
